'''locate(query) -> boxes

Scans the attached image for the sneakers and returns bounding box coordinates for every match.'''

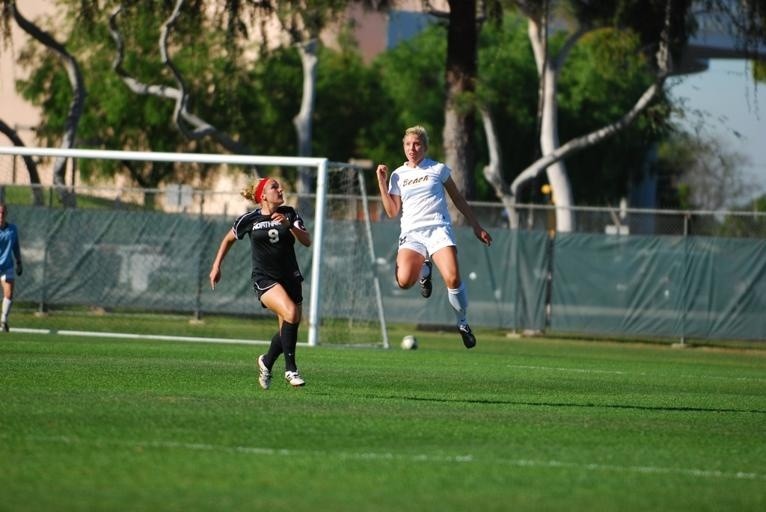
[285,369,305,386]
[457,323,476,349]
[0,322,9,332]
[258,354,271,390]
[420,260,432,298]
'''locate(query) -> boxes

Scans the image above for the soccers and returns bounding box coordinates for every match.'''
[400,334,418,352]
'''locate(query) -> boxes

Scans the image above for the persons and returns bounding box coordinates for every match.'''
[209,176,312,389]
[0,200,22,333]
[375,124,492,348]
